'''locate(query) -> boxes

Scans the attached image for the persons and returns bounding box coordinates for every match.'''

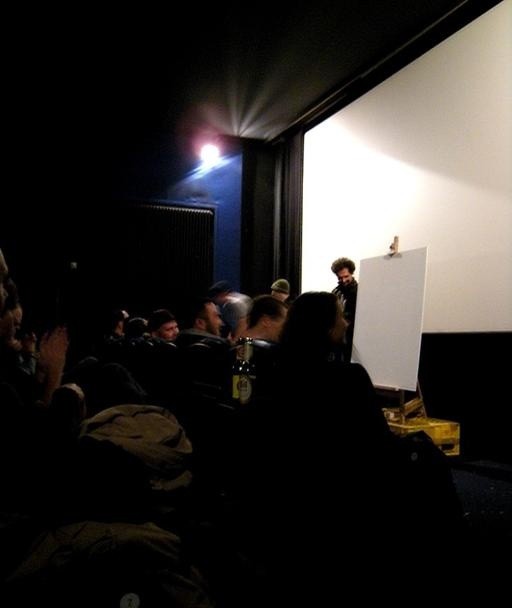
[1,248,500,602]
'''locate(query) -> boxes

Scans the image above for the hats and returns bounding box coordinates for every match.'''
[207,280,233,297]
[270,278,290,294]
[150,309,175,330]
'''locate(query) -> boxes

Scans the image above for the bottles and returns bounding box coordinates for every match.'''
[239,336,257,405]
[231,338,245,400]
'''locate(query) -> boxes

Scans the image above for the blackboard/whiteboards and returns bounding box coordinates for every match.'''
[350,246,426,391]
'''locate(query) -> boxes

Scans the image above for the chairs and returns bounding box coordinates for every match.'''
[103,335,238,419]
